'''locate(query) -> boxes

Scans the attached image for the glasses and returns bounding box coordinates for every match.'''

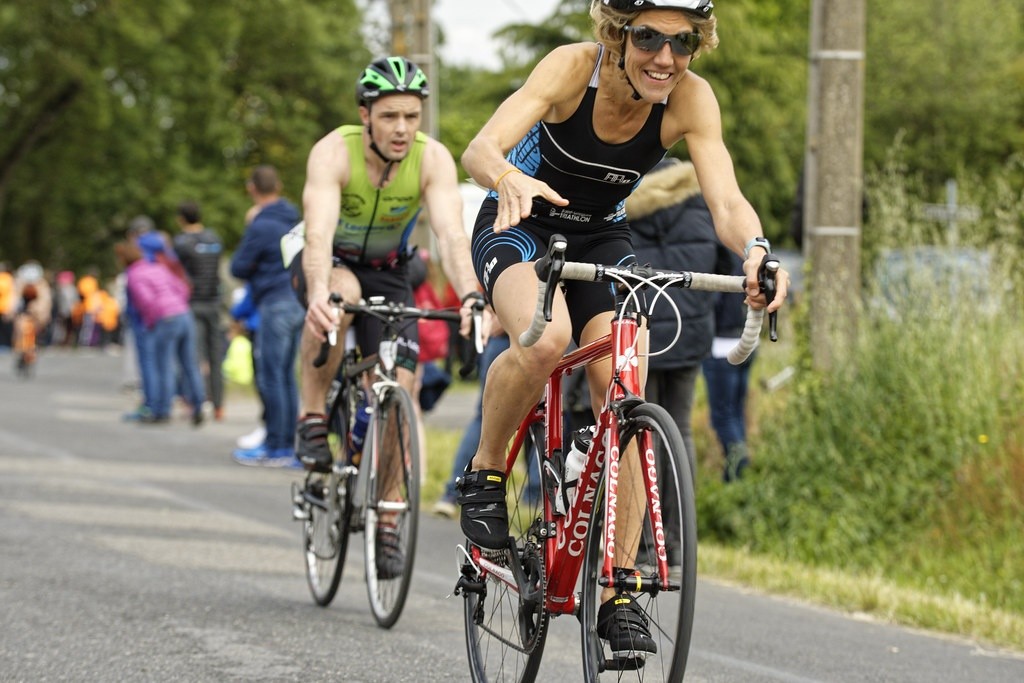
[623,25,702,55]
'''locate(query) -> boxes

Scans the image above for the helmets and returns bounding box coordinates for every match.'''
[355,57,431,104]
[602,0,714,19]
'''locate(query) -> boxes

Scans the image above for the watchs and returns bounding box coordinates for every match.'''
[743,236,771,260]
[461,291,489,306]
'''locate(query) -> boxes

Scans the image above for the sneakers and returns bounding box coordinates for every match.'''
[456,454,510,552]
[233,443,303,468]
[597,594,658,660]
[376,521,406,580]
[294,413,333,469]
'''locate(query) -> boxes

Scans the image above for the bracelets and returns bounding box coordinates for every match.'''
[494,168,520,191]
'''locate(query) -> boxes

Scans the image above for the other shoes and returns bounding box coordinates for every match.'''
[432,502,456,518]
[725,448,749,482]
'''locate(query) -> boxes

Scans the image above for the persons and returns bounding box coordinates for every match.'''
[404,154,758,521]
[456,0,789,658]
[3,164,305,469]
[281,55,493,472]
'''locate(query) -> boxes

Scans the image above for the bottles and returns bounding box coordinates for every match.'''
[351,401,372,449]
[555,425,596,515]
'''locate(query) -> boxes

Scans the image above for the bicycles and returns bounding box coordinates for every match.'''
[457,234,777,683]
[289,291,484,630]
